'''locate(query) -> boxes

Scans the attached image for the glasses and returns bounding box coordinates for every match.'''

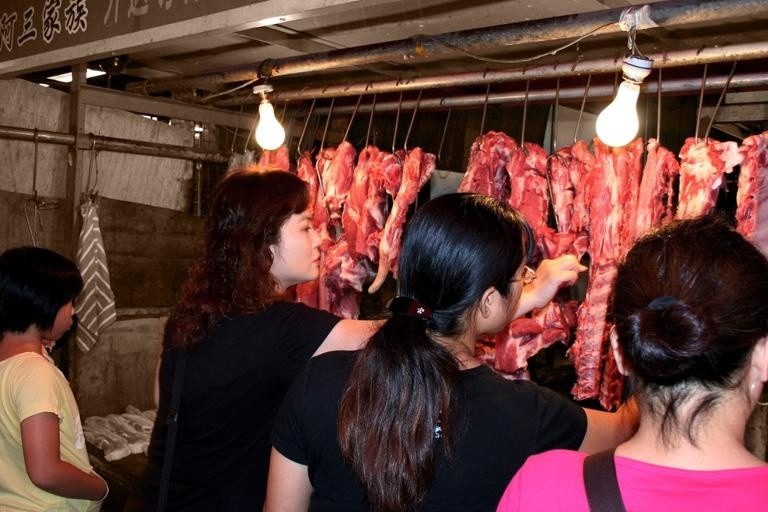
[510,265,537,284]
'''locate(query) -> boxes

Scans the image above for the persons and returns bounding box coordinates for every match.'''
[496,215,768,512]
[131,168,590,511]
[263,191,640,511]
[1,245,110,512]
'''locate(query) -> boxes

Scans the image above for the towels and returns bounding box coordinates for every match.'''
[73,200,118,354]
